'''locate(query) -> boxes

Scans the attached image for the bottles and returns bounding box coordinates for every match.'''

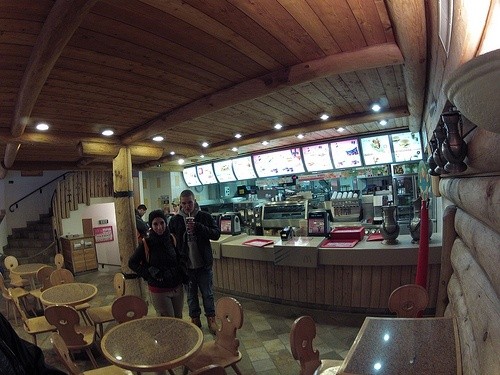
[381,201,399,245]
[410,195,434,244]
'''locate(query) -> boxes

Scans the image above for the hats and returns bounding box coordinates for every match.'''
[148,210,167,228]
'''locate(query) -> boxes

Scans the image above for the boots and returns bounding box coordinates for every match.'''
[208,316,219,332]
[192,316,201,328]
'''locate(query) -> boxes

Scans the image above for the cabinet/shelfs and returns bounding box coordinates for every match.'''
[60,235,98,279]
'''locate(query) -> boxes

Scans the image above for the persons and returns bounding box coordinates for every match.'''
[128,208,185,320]
[167,190,220,336]
[172,196,181,215]
[135,205,150,239]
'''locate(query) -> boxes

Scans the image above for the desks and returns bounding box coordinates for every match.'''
[39,282,98,310]
[10,263,48,310]
[337,317,463,375]
[101,317,204,375]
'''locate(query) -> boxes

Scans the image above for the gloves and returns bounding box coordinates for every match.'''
[184,271,190,285]
[148,267,163,282]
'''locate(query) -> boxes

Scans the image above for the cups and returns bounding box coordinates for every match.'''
[186,217,195,234]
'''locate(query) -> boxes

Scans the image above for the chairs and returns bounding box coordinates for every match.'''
[388,284,429,317]
[290,315,344,375]
[0,254,245,375]
